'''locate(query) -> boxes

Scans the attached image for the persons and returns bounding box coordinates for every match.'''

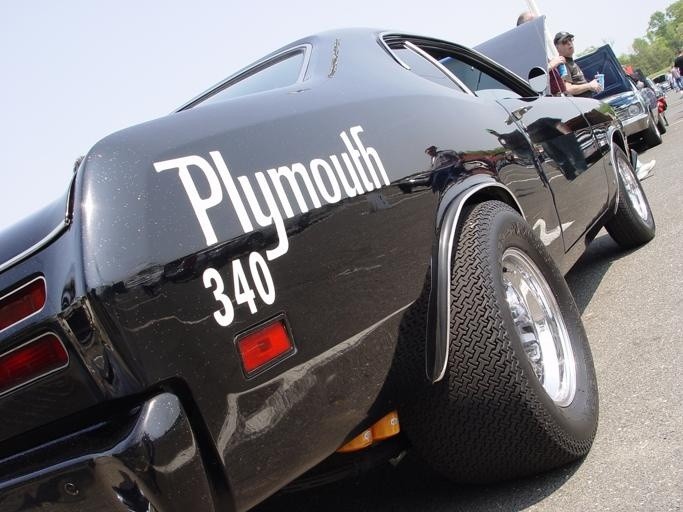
[425,145,463,194]
[524,118,588,183]
[552,30,656,180]
[665,49,683,93]
[516,12,566,96]
[621,64,669,127]
[485,128,527,153]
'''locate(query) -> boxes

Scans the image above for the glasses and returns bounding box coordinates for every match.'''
[562,36,573,45]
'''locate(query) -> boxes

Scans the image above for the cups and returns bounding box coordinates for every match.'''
[594,74,605,91]
[557,62,569,78]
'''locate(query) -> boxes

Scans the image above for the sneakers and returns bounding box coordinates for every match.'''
[635,159,656,181]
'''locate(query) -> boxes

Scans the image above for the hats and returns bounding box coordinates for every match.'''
[553,31,574,45]
